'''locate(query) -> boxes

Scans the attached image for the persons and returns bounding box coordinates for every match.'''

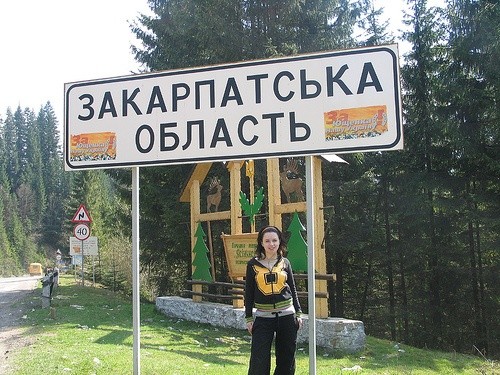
[244,226,303,375]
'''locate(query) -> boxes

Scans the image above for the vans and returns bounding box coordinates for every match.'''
[28,263,42,277]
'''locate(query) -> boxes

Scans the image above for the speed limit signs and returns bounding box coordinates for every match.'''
[73,223,91,240]
